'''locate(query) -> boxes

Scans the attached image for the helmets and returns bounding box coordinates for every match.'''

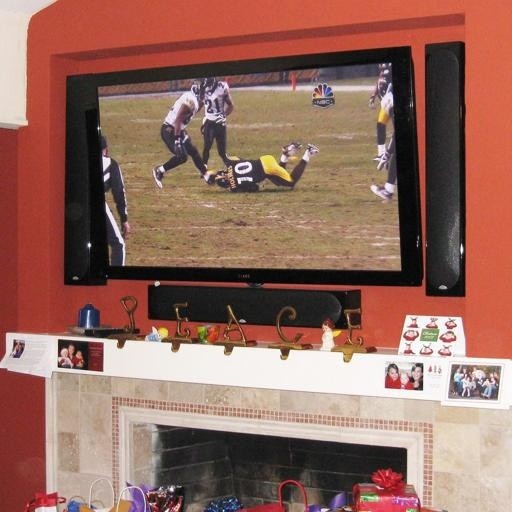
[215,169,229,188]
[381,63,392,84]
[191,77,217,97]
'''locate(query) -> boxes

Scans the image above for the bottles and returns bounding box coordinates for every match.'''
[79,303,99,327]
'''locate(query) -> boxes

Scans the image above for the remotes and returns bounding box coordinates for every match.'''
[84,327,141,340]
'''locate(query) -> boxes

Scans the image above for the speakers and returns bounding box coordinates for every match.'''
[148,285,362,331]
[423,40,466,296]
[63,74,103,288]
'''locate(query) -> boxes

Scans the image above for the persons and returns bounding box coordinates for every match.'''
[453,365,499,399]
[151,80,213,188]
[99,134,130,266]
[319,318,336,351]
[199,76,235,176]
[214,141,319,192]
[385,364,401,388]
[369,64,394,163]
[404,364,423,390]
[369,80,398,201]
[58,344,85,368]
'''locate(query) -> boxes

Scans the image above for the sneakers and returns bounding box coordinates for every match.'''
[206,171,214,185]
[152,165,163,189]
[370,184,394,200]
[281,141,302,156]
[306,143,320,154]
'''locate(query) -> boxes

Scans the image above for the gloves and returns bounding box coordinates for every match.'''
[214,112,227,126]
[374,151,392,170]
[369,96,375,108]
[175,136,183,153]
[182,131,190,144]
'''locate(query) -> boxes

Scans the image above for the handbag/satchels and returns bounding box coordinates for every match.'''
[25,478,148,512]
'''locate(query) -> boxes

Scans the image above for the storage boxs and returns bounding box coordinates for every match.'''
[352,483,420,512]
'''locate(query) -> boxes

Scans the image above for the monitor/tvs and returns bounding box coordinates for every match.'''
[98,44,424,286]
